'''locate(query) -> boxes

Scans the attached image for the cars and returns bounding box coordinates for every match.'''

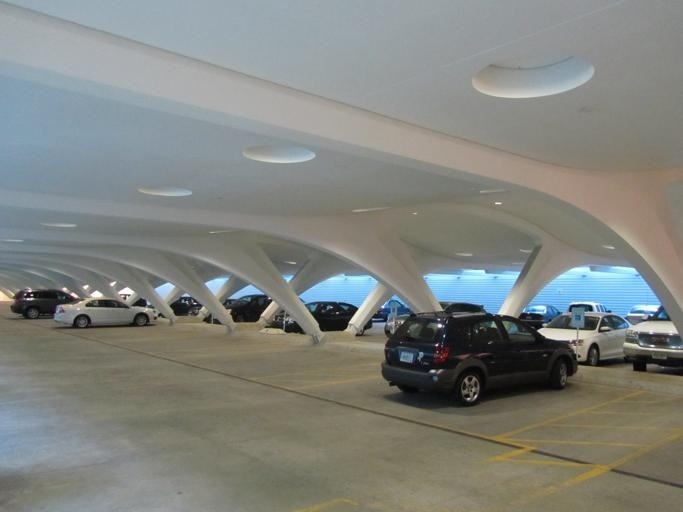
[9,287,161,328]
[381,311,577,406]
